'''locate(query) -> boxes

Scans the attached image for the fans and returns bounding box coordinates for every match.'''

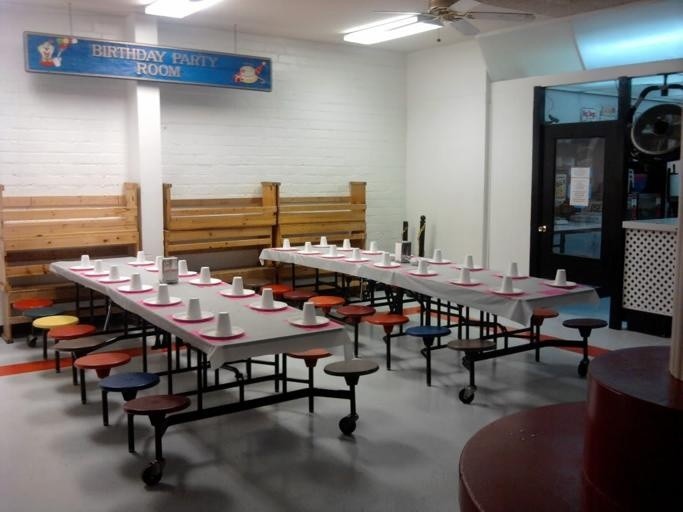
[373,0,537,38]
[631,104,682,161]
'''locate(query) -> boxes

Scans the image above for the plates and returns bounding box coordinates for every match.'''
[411,269,438,276]
[497,273,527,278]
[98,276,131,283]
[335,247,360,251]
[144,267,159,271]
[272,247,298,251]
[200,325,244,337]
[144,297,181,305]
[343,258,368,262]
[219,288,256,297]
[297,250,320,255]
[249,301,288,310]
[452,278,480,286]
[320,254,346,258]
[544,280,577,286]
[173,311,214,321]
[361,251,385,254]
[373,262,401,268]
[427,259,449,264]
[84,271,110,276]
[118,285,152,292]
[455,265,483,270]
[288,315,330,326]
[69,267,95,270]
[313,245,336,248]
[178,271,197,275]
[128,260,154,265]
[492,287,523,294]
[189,278,222,285]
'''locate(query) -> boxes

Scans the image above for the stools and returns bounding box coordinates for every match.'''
[308,295,346,317]
[337,305,376,357]
[259,283,292,301]
[323,359,379,436]
[282,290,317,310]
[367,314,409,370]
[244,278,270,294]
[562,318,608,377]
[98,371,159,452]
[55,336,106,386]
[11,298,53,346]
[124,394,191,460]
[286,353,334,413]
[447,339,497,406]
[405,325,450,386]
[23,307,66,359]
[47,324,96,386]
[33,315,78,373]
[532,309,559,363]
[73,351,132,426]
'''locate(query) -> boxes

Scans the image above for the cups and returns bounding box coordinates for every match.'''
[459,268,470,284]
[467,255,473,268]
[188,298,201,318]
[353,248,361,260]
[370,241,378,251]
[508,263,518,276]
[200,267,210,283]
[433,249,442,262]
[418,260,428,273]
[178,260,188,275]
[329,245,337,256]
[305,242,311,252]
[155,256,163,270]
[216,312,232,336]
[261,288,273,308]
[302,302,315,325]
[343,239,351,249]
[554,269,566,285]
[500,276,512,292]
[131,273,141,290]
[81,254,90,268]
[158,284,169,303]
[94,261,104,274]
[283,239,290,249]
[320,237,327,246]
[383,252,390,265]
[110,265,119,279]
[136,251,145,264]
[232,277,244,294]
